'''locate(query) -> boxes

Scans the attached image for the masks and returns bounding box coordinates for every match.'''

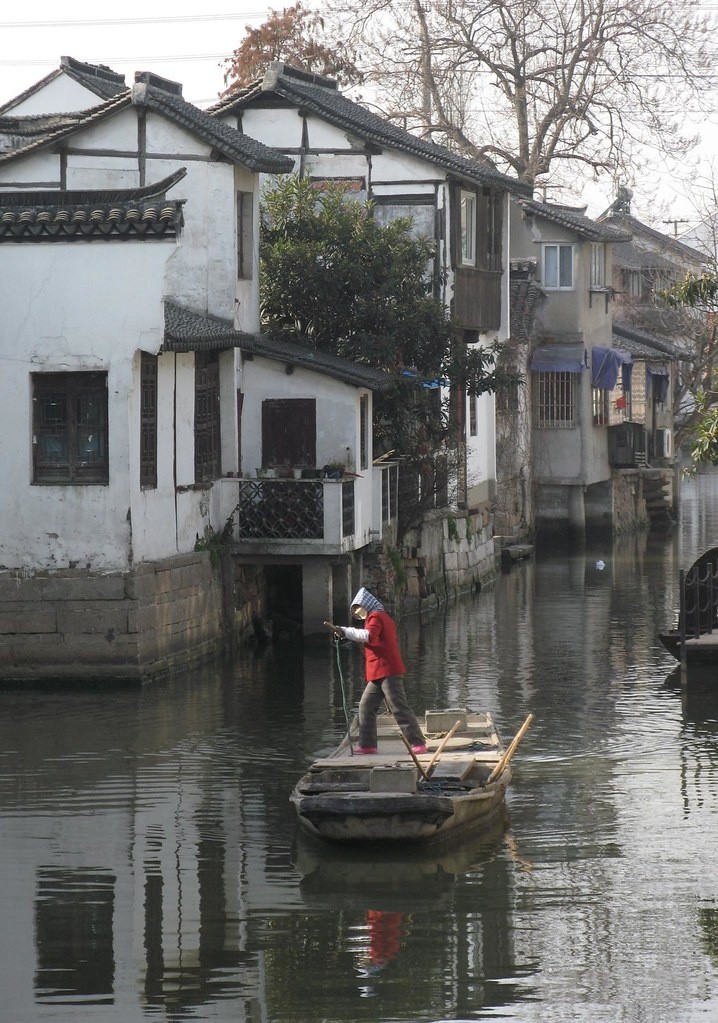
[354,607,368,619]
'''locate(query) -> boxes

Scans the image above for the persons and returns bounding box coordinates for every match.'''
[335,585,429,756]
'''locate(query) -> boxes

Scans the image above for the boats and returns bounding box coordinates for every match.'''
[656,546,717,661]
[288,704,536,847]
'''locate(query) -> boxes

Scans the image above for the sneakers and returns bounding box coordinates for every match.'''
[408,744,427,754]
[353,746,377,754]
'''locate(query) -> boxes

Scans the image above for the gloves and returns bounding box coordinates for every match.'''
[334,626,370,643]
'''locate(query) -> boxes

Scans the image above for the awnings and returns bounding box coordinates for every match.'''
[530,344,586,374]
[646,365,669,402]
[590,343,634,395]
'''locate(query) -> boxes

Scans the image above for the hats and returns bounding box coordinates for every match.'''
[349,586,384,620]
